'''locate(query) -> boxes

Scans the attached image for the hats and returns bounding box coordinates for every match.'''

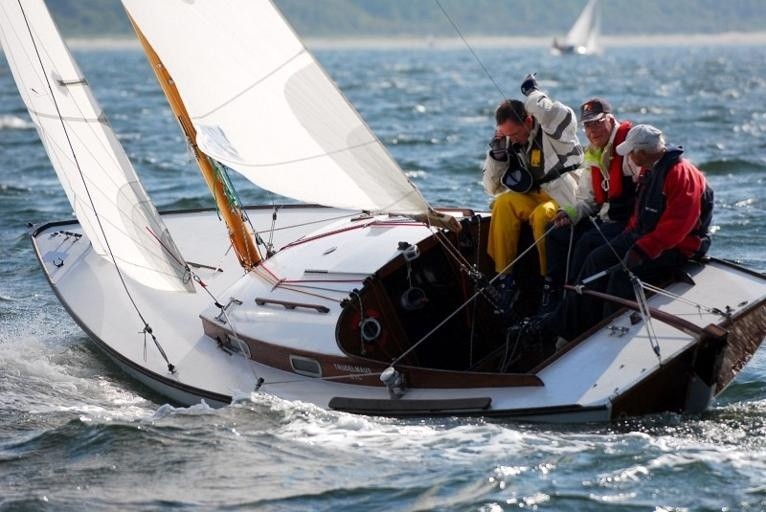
[579,98,611,123]
[615,123,662,156]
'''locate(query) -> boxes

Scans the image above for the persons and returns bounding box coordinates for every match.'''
[479,71,586,308]
[570,125,711,348]
[543,97,637,296]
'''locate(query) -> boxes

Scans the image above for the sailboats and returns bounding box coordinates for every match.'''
[0,0,765,429]
[551,0,605,56]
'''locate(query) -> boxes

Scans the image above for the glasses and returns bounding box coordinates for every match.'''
[582,118,608,129]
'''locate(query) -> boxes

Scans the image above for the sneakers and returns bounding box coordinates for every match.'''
[492,278,521,315]
[536,275,567,319]
